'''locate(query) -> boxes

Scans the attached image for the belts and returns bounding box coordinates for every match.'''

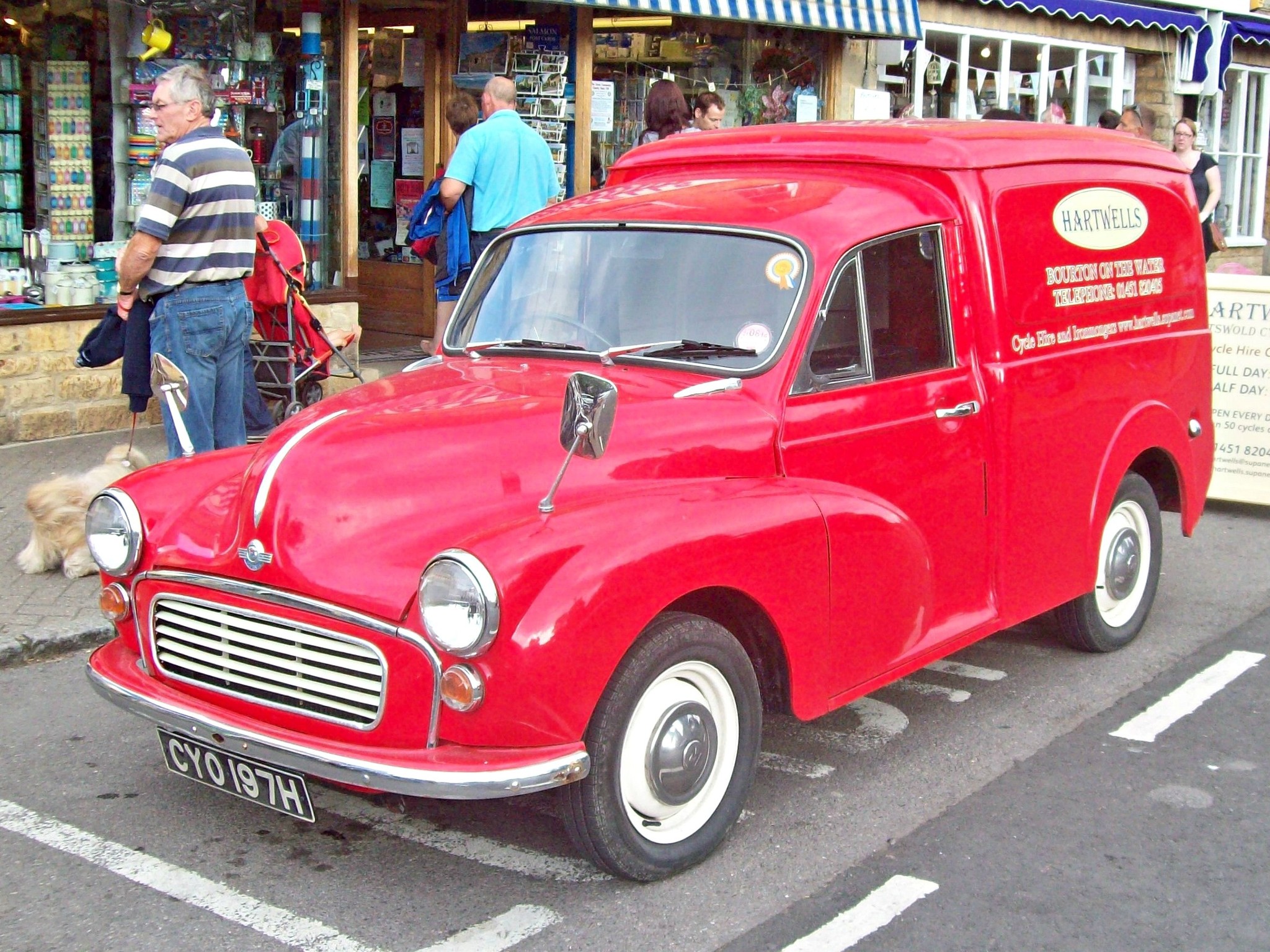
[165,282,205,295]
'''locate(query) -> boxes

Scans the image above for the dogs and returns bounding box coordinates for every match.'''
[14,446,150,579]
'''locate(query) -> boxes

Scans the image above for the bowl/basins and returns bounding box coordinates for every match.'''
[89,258,119,297]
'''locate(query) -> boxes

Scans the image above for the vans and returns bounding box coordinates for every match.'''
[85,122,1212,882]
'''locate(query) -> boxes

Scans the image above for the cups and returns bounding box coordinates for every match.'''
[44,271,101,307]
[233,42,251,61]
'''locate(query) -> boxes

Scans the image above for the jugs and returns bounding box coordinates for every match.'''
[139,19,171,63]
[250,31,282,61]
[210,71,226,91]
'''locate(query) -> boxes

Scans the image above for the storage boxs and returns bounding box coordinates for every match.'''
[594,33,687,61]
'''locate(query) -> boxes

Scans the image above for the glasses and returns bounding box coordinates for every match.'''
[1175,132,1194,139]
[147,99,205,111]
[1131,104,1144,127]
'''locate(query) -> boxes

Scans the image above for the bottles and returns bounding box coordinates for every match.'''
[249,126,268,164]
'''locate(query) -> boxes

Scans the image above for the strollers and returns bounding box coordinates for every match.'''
[246,218,369,426]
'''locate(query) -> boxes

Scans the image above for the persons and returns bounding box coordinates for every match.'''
[982,102,1158,141]
[1171,117,1220,264]
[269,81,343,227]
[633,79,726,149]
[421,76,562,356]
[117,65,275,462]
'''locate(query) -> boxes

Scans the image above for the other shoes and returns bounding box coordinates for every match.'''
[420,340,433,356]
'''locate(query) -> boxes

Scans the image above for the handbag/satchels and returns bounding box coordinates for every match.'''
[1208,222,1226,252]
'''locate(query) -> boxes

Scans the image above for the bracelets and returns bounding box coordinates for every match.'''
[119,290,135,295]
[118,302,131,312]
